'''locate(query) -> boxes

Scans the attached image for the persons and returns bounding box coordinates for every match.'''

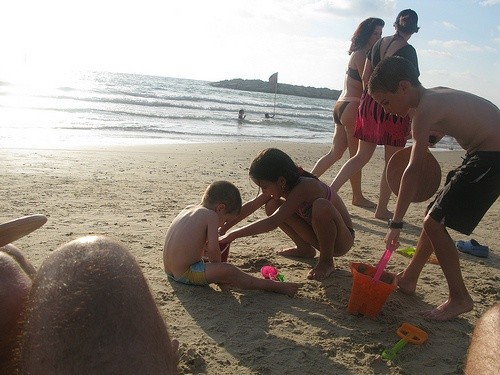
[329,9,420,219]
[367,56,500,320]
[238,110,246,119]
[205,147,355,280]
[163,181,300,297]
[310,18,385,208]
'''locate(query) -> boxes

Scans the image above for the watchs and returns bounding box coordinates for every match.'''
[388,219,403,229]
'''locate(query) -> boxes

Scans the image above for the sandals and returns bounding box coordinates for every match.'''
[455,238,489,257]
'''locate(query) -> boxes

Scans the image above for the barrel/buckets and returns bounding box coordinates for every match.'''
[348,262,397,318]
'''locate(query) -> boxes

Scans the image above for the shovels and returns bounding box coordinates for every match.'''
[382,322,428,360]
[261,266,284,282]
